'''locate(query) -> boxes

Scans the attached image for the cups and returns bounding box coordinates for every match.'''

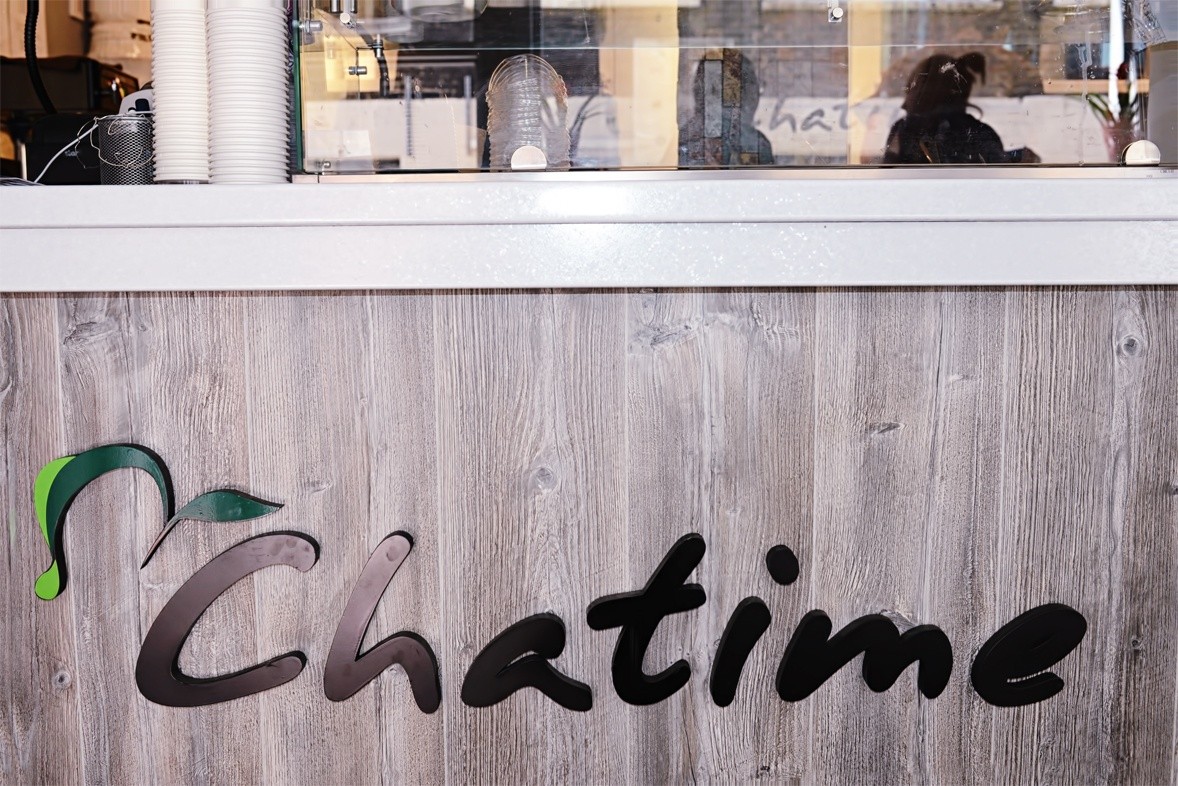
[150,0,289,184]
